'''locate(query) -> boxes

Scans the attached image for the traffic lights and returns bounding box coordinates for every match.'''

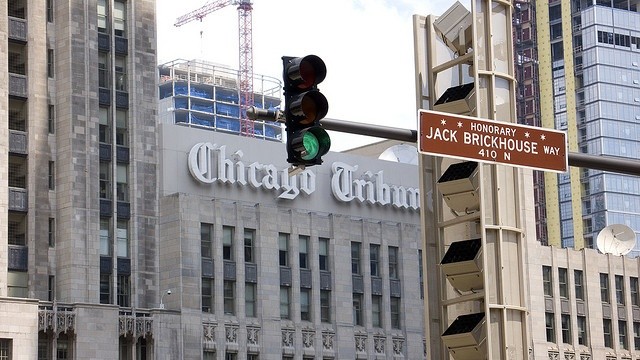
[281,54,331,167]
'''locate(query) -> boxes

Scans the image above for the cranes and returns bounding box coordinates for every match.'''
[175,1,257,136]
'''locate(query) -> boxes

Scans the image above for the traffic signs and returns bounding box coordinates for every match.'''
[417,108,568,175]
